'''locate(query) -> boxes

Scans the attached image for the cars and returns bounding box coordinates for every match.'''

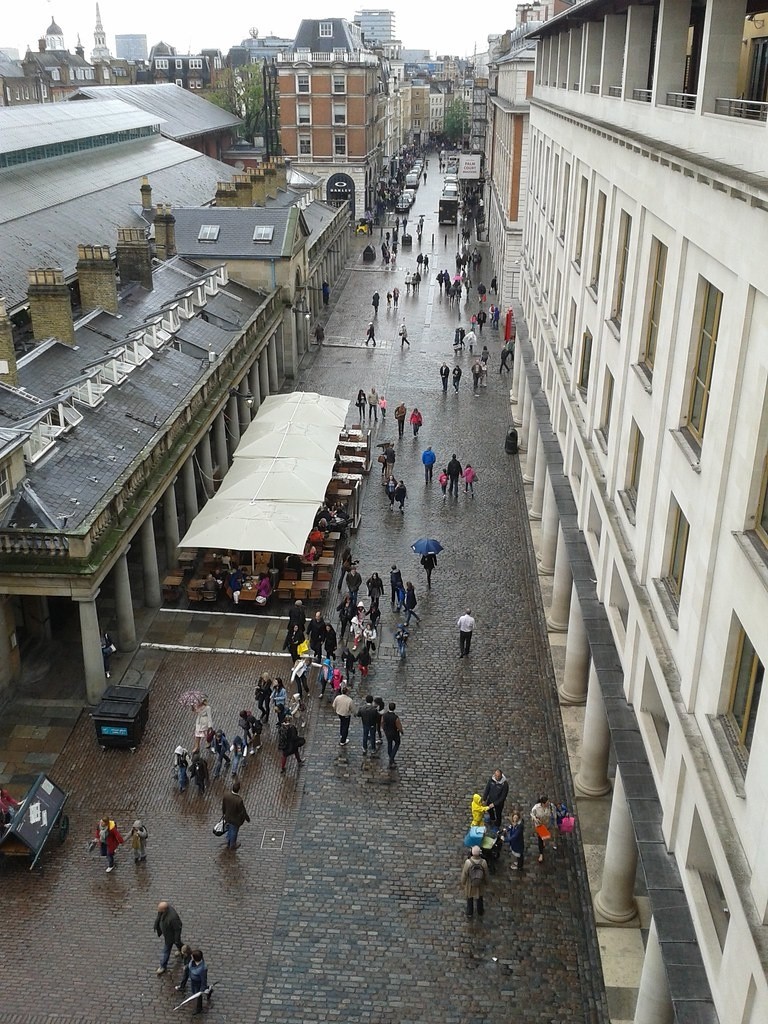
[395,189,416,214]
[406,158,424,189]
[442,156,459,196]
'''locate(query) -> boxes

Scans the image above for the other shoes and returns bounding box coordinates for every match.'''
[207,989,213,999]
[106,865,115,872]
[263,717,268,723]
[511,862,518,869]
[340,739,349,745]
[229,842,241,850]
[175,986,185,990]
[157,967,165,973]
[300,758,306,763]
[193,1008,203,1015]
[260,714,265,718]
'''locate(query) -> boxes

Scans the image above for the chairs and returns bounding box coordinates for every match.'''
[162,423,372,611]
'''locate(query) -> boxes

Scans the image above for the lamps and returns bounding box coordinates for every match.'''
[228,387,255,409]
[293,307,312,321]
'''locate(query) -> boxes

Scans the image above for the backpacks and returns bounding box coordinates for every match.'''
[469,858,485,887]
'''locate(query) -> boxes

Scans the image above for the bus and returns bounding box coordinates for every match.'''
[438,196,461,226]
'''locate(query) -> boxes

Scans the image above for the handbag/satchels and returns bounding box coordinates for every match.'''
[296,737,305,747]
[213,816,229,837]
[535,824,551,840]
[561,814,575,832]
[297,639,310,655]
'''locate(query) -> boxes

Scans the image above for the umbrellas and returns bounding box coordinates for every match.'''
[411,537,444,555]
[173,981,220,1011]
[178,690,208,706]
[452,275,463,282]
[176,391,352,571]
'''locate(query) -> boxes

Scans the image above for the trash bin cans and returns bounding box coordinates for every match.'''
[505,428,517,454]
[93,686,149,748]
[363,243,376,261]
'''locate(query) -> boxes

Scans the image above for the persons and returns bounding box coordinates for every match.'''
[94,816,148,873]
[332,686,404,767]
[377,442,409,515]
[314,134,515,394]
[203,564,272,609]
[357,388,423,439]
[0,789,24,830]
[457,608,475,659]
[153,901,214,1015]
[173,672,306,797]
[283,494,438,702]
[222,781,250,849]
[460,768,575,919]
[422,444,476,499]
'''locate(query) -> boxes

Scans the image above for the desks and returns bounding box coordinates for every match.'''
[276,578,332,590]
[347,473,363,481]
[321,530,341,542]
[345,441,368,450]
[178,551,198,567]
[353,456,366,463]
[338,440,346,446]
[238,574,261,601]
[340,429,346,435]
[284,556,335,569]
[189,578,224,590]
[162,575,184,592]
[332,471,348,480]
[330,487,352,506]
[347,428,364,439]
[204,553,235,562]
[341,454,352,464]
